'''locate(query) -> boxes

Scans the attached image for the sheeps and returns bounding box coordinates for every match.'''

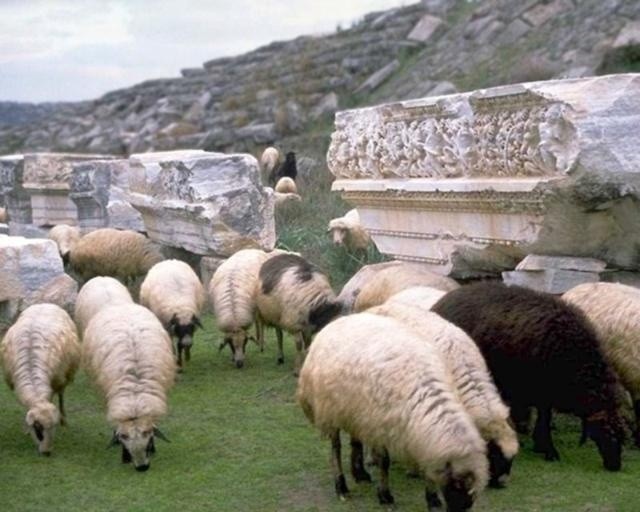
[260,146,303,207]
[207,248,345,378]
[325,208,372,269]
[295,264,640,512]
[0,224,206,472]
